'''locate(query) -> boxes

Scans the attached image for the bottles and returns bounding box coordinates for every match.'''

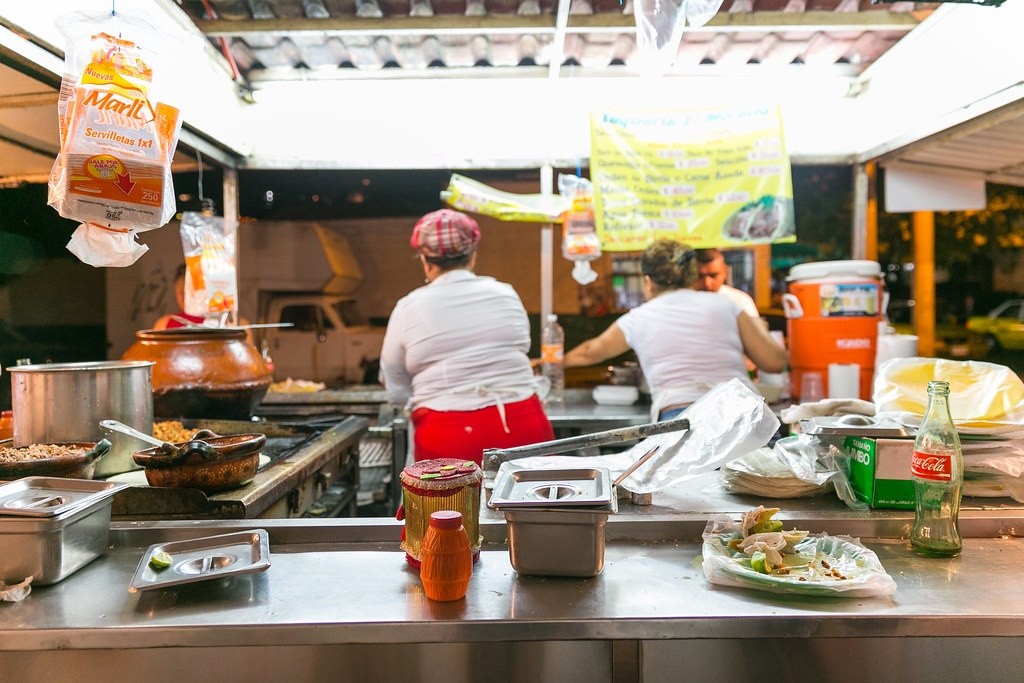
[910,381,965,557]
[0,410,13,440]
[540,313,565,405]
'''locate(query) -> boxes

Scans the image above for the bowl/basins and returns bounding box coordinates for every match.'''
[592,385,639,406]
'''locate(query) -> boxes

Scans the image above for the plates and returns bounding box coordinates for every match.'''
[709,533,874,596]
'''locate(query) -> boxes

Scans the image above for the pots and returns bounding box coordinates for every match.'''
[133,428,266,492]
[5,360,158,478]
[121,328,271,422]
[0,438,112,481]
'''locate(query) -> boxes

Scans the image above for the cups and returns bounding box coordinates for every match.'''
[799,373,823,406]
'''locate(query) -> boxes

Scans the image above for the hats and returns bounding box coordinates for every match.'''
[409,208,480,257]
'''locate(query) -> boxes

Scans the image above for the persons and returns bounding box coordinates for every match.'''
[377,209,556,471]
[152,260,256,352]
[683,244,772,389]
[529,235,791,425]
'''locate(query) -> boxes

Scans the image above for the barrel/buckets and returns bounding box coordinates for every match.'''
[782,259,885,403]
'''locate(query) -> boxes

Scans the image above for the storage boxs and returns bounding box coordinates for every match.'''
[841,435,942,512]
